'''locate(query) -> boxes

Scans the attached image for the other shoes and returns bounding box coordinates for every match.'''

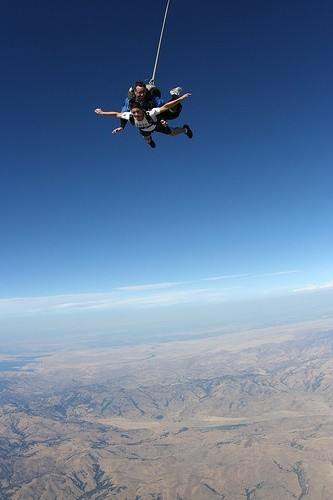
[147,136,156,148]
[183,124,193,138]
[170,86,182,95]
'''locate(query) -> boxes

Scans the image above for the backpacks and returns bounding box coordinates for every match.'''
[128,81,162,103]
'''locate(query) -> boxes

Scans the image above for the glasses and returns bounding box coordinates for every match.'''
[135,89,147,93]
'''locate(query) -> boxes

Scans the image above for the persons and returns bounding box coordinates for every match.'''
[112,81,185,134]
[94,92,193,148]
